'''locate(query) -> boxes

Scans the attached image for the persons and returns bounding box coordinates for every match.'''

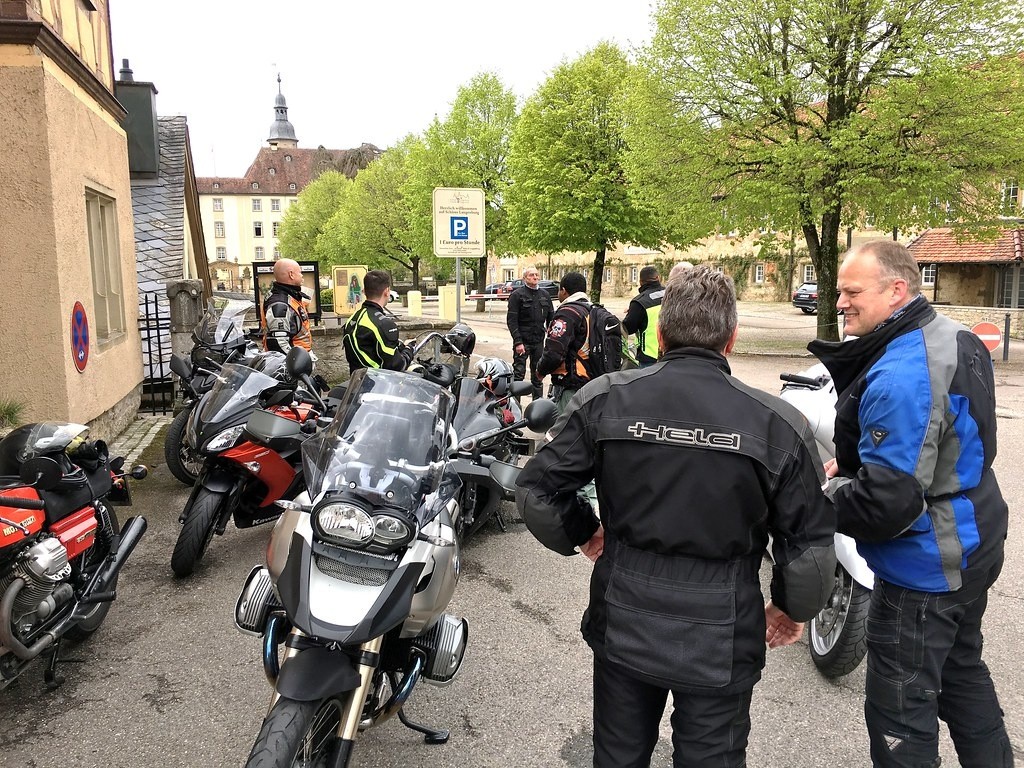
[342,271,417,379]
[823,240,1014,768]
[516,267,835,768]
[262,258,316,378]
[507,266,555,403]
[622,266,670,366]
[535,272,599,515]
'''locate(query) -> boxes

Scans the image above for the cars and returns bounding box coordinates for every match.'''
[476,283,503,301]
[503,279,524,293]
[792,280,843,316]
[539,280,560,298]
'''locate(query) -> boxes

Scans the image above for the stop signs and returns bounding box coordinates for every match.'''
[971,322,1001,352]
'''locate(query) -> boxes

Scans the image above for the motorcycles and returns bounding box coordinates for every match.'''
[773,362,875,680]
[0,420,148,695]
[163,295,563,578]
[235,346,560,768]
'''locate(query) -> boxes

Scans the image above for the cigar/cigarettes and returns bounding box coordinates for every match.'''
[519,353,522,356]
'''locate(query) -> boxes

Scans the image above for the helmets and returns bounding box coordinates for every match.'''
[474,357,514,395]
[0,421,89,491]
[446,322,476,355]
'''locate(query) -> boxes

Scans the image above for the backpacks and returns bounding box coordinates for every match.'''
[565,300,622,380]
